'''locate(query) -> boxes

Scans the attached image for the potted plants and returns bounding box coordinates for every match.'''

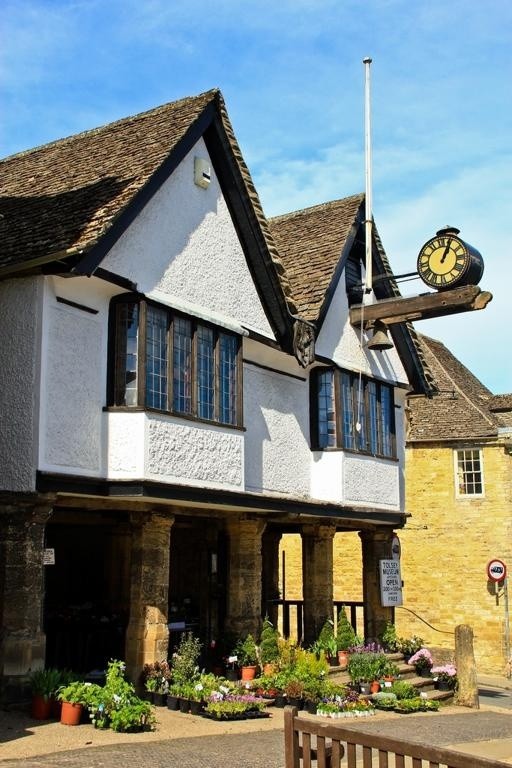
[139,630,269,720]
[382,615,457,691]
[27,655,155,732]
[317,608,442,715]
[226,618,377,719]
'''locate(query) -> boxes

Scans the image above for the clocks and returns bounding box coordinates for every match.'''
[417,227,484,292]
